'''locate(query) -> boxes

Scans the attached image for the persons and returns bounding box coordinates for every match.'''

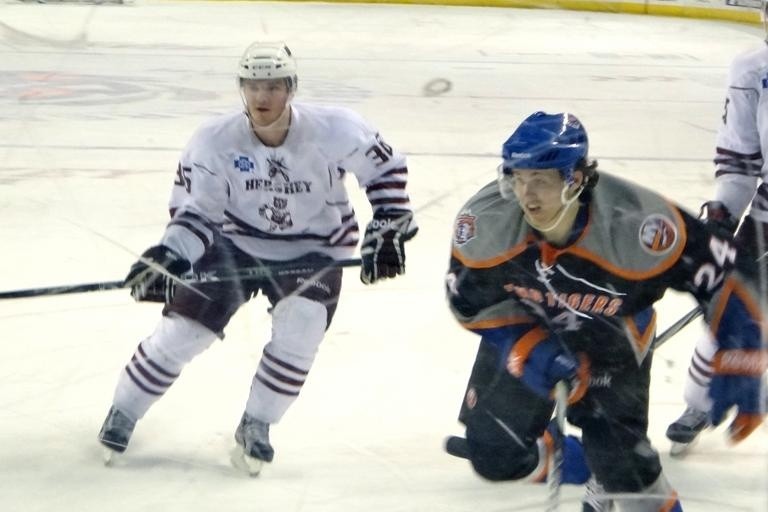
[97,40,410,464]
[443,110,766,512]
[665,2,767,445]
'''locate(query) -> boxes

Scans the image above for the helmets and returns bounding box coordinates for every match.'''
[501,111,586,200]
[238,41,296,96]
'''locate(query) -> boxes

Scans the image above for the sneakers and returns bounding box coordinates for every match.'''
[668,406,706,444]
[584,474,615,512]
[235,413,273,461]
[98,407,135,454]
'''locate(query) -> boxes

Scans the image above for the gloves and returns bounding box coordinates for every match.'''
[699,200,756,255]
[706,337,766,441]
[359,205,414,283]
[124,244,192,305]
[504,327,587,403]
